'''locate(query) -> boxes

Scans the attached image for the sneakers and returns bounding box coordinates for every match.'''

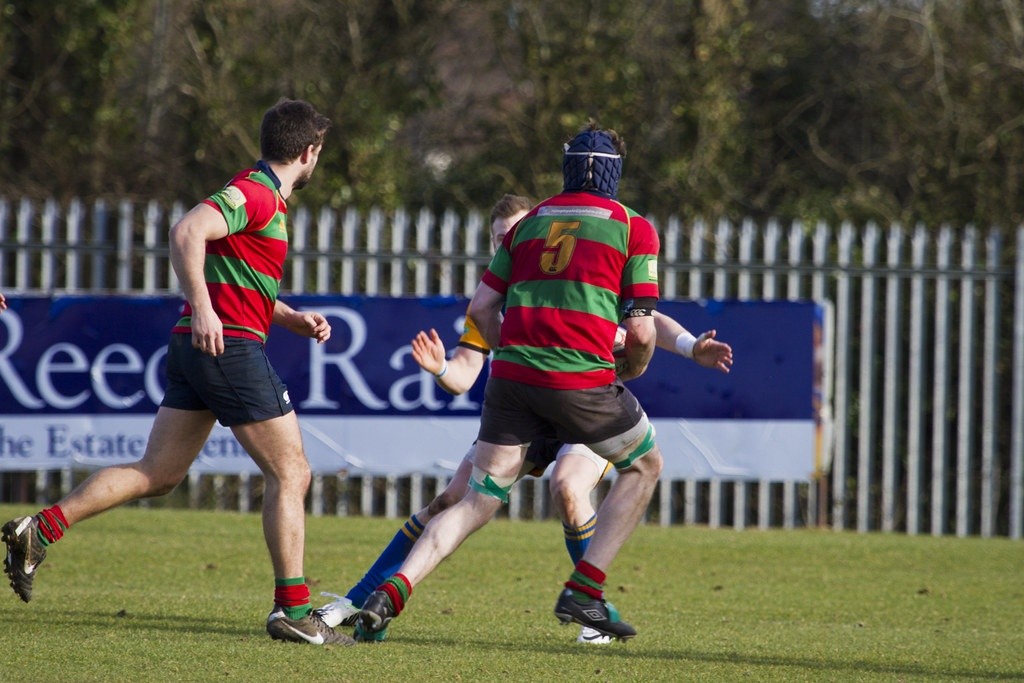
[1,516,47,603]
[353,591,394,643]
[266,599,356,645]
[314,592,361,628]
[554,588,637,643]
[577,626,616,644]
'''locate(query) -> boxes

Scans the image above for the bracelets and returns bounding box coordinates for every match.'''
[674,331,697,359]
[435,363,447,378]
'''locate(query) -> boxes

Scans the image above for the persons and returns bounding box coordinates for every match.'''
[1,98,358,647]
[315,123,734,644]
[0,294,7,313]
